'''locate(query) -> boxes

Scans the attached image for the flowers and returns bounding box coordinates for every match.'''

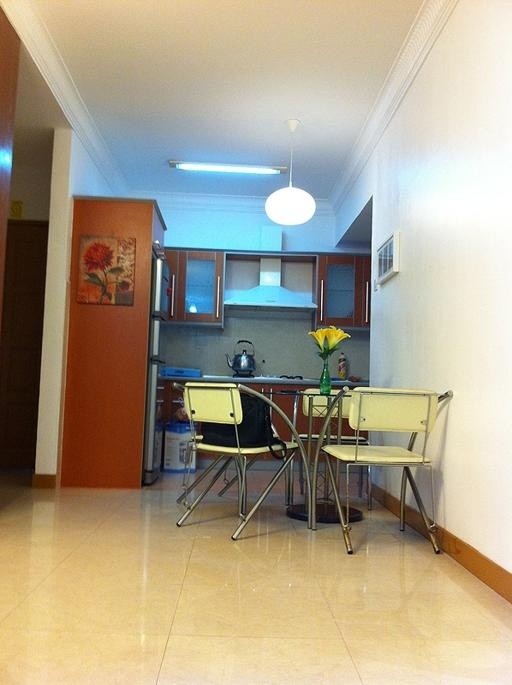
[307,325,351,364]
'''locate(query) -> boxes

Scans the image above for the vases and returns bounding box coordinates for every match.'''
[320,363,331,394]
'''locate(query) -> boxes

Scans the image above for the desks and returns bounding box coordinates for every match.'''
[259,387,363,524]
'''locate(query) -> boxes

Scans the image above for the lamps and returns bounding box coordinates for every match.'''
[264,119,316,226]
[168,160,288,175]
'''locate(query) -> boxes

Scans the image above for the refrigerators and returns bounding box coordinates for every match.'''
[143,255,177,488]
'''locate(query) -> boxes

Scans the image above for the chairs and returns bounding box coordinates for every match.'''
[290,388,374,510]
[171,379,312,541]
[312,386,453,554]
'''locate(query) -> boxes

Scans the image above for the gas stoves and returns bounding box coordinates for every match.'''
[203,375,321,385]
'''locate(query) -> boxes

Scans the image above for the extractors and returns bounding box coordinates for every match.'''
[224,257,318,312]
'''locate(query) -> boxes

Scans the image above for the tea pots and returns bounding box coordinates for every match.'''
[225,339,256,371]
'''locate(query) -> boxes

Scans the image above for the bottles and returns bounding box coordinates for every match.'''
[338,352,348,380]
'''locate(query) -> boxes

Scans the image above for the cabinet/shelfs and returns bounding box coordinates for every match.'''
[167,249,224,323]
[317,255,370,327]
[246,383,368,462]
